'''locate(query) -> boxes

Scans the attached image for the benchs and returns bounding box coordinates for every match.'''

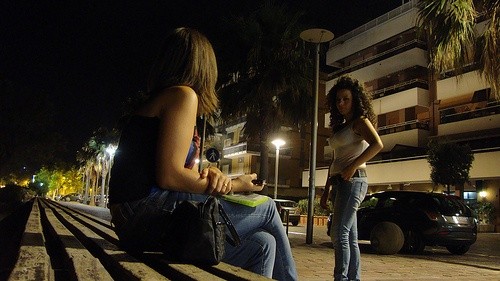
[9,197,276,281]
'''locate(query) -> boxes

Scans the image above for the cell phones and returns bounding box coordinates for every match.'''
[252,180,262,186]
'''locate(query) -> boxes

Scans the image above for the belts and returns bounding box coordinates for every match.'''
[329,168,359,184]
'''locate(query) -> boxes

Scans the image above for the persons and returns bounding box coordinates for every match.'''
[107,26,299,281]
[320,77,383,281]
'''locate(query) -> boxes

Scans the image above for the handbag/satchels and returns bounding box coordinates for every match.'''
[163,196,239,266]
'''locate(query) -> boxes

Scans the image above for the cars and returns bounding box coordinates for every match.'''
[327,191,477,253]
[272,199,300,226]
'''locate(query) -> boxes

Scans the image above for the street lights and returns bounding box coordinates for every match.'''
[300,26,334,244]
[272,140,285,199]
